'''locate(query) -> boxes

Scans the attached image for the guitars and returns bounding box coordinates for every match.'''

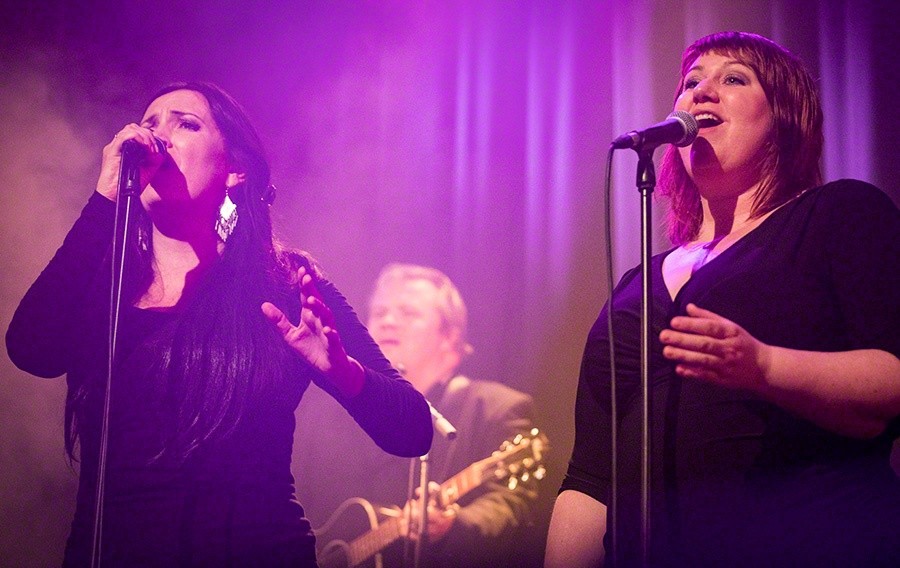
[310,426,553,568]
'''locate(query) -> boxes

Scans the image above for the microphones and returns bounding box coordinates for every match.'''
[612,109,700,149]
[120,136,167,165]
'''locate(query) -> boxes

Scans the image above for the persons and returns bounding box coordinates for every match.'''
[6,79,434,568]
[542,28,899,568]
[304,261,548,568]
